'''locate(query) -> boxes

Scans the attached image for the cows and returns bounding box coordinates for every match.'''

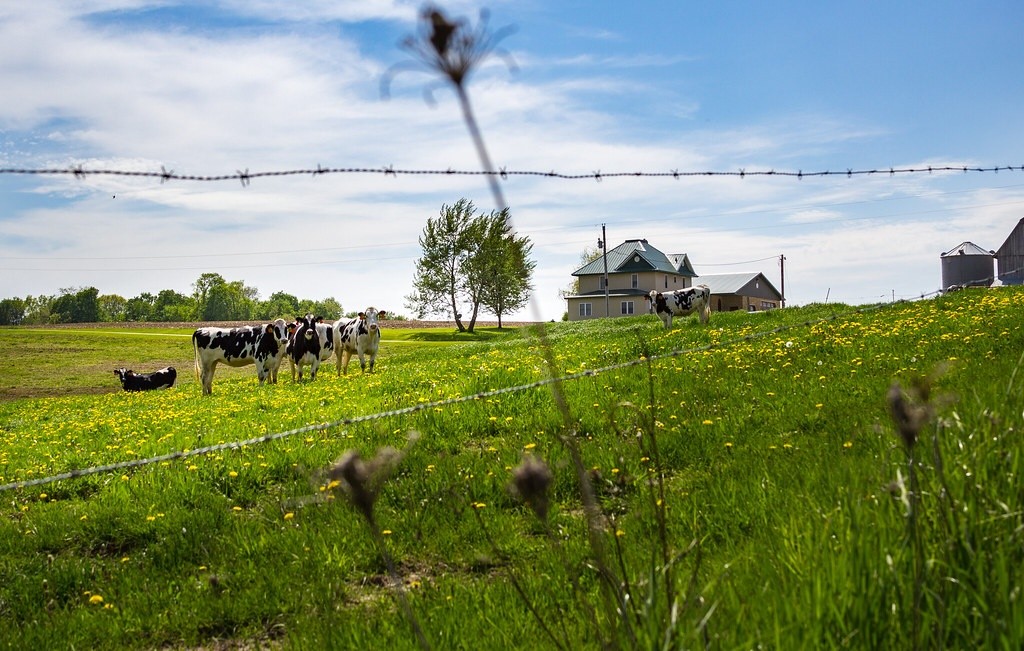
[113,366,178,392]
[191,307,386,396]
[644,284,710,328]
